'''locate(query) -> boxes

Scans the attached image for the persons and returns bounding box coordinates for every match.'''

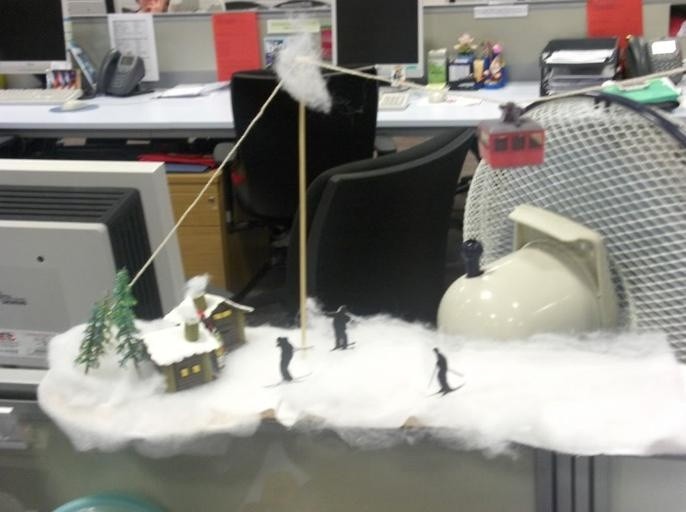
[134,1,169,15]
[322,303,352,349]
[462,231,485,279]
[274,335,294,382]
[432,345,452,395]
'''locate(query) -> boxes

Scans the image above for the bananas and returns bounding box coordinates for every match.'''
[378,91,410,110]
[0,88,83,105]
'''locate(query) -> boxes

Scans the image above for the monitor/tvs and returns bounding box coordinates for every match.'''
[602,76,682,104]
[653,100,680,111]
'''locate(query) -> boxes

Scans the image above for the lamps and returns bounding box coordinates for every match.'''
[222,64,380,296]
[284,121,476,330]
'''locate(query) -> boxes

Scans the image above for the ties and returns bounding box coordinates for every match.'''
[0,0,72,88]
[0,157,188,398]
[331,0,425,92]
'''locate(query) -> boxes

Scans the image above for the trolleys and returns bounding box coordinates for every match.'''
[435,91,686,365]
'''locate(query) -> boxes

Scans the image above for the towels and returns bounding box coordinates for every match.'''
[57,492,164,512]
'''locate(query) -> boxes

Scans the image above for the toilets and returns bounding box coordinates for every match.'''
[622,34,683,86]
[96,49,145,96]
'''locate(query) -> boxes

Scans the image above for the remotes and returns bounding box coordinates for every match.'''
[62,100,89,111]
[428,91,446,103]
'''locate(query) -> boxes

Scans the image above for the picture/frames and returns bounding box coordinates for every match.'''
[0,1,645,139]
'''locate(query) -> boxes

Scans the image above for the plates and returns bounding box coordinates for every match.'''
[165,162,266,298]
[539,34,623,101]
